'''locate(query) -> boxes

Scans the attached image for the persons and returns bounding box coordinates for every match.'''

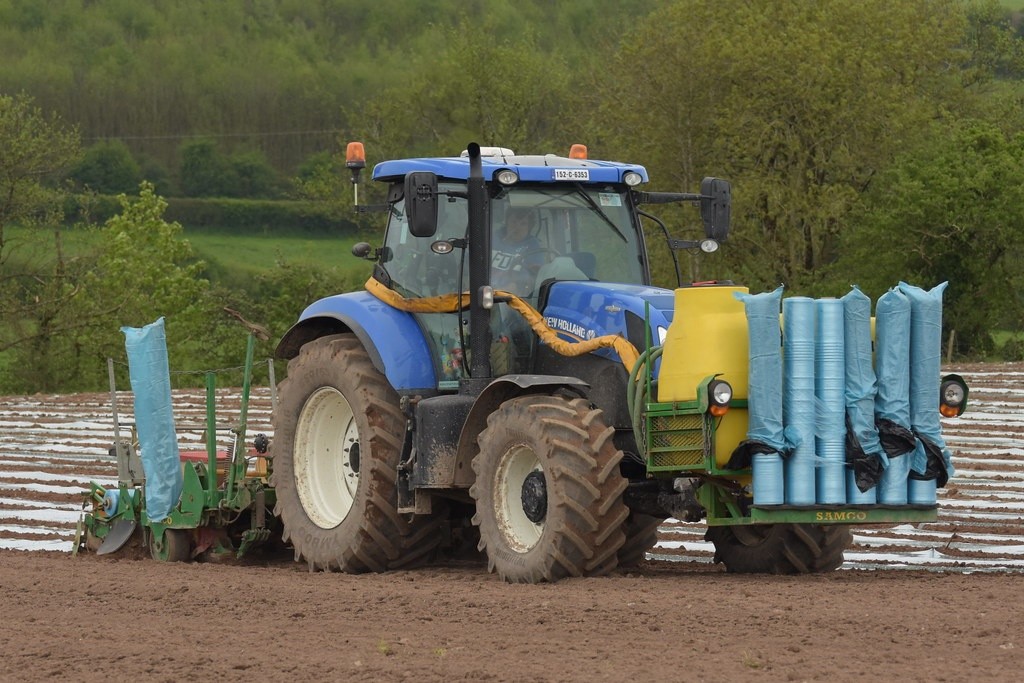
[492,204,544,299]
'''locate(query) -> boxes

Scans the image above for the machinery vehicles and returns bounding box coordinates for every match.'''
[71,139,971,584]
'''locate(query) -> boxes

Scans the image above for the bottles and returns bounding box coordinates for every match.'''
[442,341,463,381]
